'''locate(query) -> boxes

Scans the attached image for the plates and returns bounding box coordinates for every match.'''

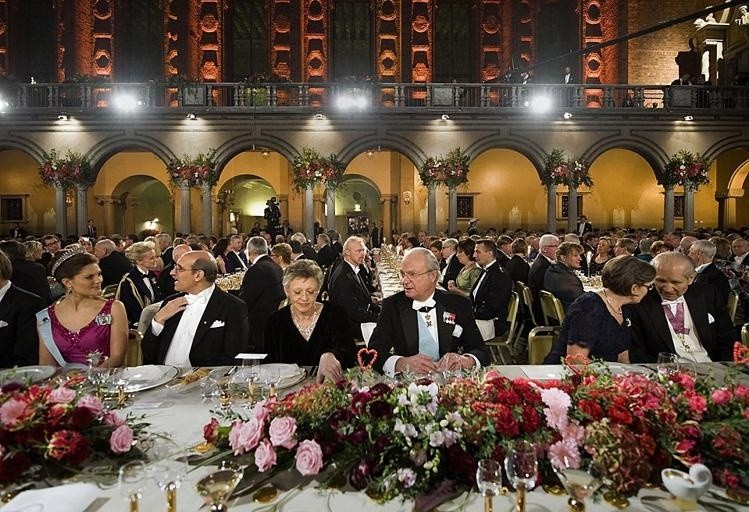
[230,363,307,392]
[0,362,56,388]
[99,364,180,394]
[584,361,642,377]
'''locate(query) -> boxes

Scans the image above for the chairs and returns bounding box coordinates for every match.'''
[101,284,118,301]
[513,280,543,349]
[124,327,145,367]
[484,291,519,365]
[539,290,565,328]
[528,326,560,365]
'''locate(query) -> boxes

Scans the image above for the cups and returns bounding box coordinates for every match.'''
[655,350,679,377]
[224,266,242,281]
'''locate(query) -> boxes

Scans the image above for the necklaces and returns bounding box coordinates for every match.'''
[603,289,624,315]
[288,304,321,336]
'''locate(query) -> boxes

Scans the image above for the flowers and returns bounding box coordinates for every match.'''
[540,147,566,185]
[562,157,595,191]
[165,154,198,188]
[191,146,219,186]
[657,147,709,193]
[417,157,445,186]
[439,147,470,188]
[61,150,91,185]
[287,148,326,198]
[319,152,347,185]
[39,148,71,189]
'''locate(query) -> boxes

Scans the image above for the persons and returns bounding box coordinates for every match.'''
[0,194,749,388]
[669,71,694,85]
[557,64,576,107]
[519,69,535,83]
[540,254,658,364]
[250,259,354,386]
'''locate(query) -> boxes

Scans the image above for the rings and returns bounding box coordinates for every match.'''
[337,371,342,376]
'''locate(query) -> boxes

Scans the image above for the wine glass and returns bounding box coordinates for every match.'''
[118,442,190,512]
[241,357,262,403]
[263,366,281,400]
[475,438,539,512]
[548,455,608,512]
[191,460,245,511]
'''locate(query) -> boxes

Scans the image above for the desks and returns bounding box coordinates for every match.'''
[371,246,449,306]
[574,272,603,293]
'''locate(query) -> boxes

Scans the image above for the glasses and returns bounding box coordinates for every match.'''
[46,241,60,247]
[400,269,438,279]
[643,284,654,291]
[174,264,207,277]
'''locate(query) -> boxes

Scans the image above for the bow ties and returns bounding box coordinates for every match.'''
[184,295,205,304]
[141,274,152,279]
[660,297,685,305]
[412,299,436,311]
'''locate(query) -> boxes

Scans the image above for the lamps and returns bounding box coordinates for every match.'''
[64,191,75,207]
[402,189,413,206]
